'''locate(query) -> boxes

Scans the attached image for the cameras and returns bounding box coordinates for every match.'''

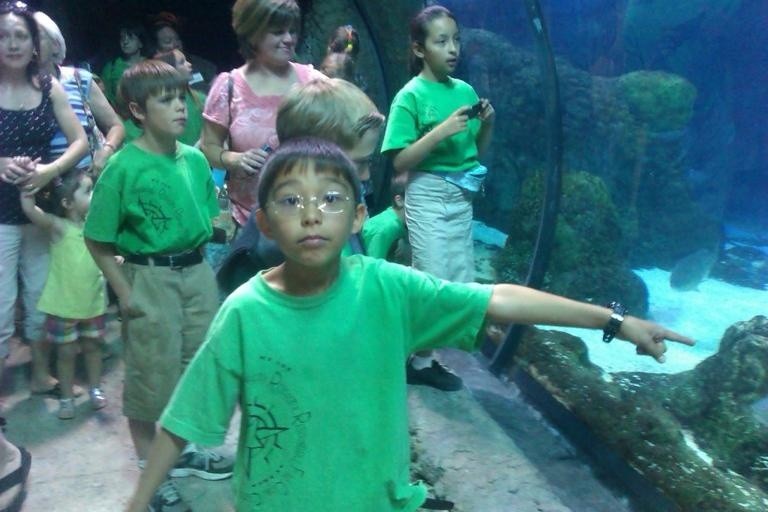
[462,102,485,118]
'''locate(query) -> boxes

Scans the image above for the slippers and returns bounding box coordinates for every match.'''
[30,381,85,400]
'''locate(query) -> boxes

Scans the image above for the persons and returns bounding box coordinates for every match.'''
[83,61,238,510]
[125,137,695,512]
[360,172,428,262]
[328,25,368,93]
[215,80,384,294]
[120,50,215,150]
[382,5,495,391]
[9,12,124,180]
[98,28,164,116]
[16,156,123,420]
[145,19,218,92]
[319,53,354,80]
[202,0,334,245]
[0,432,31,512]
[0,8,88,428]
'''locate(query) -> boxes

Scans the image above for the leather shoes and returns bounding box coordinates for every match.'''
[89,393,107,412]
[58,405,75,421]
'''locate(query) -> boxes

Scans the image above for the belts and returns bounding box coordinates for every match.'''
[124,254,205,267]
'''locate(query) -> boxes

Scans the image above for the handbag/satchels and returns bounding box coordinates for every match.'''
[216,71,233,211]
[73,65,110,181]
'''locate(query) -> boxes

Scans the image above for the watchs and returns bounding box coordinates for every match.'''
[603,300,626,342]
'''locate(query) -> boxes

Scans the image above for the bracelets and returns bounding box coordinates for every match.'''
[103,142,118,159]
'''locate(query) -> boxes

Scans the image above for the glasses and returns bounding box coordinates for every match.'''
[1,1,30,14]
[261,191,353,214]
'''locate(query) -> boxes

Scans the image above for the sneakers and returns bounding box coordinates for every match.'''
[406,354,464,392]
[168,454,238,483]
[150,485,190,512]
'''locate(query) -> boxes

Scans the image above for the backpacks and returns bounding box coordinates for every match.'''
[216,208,258,288]
